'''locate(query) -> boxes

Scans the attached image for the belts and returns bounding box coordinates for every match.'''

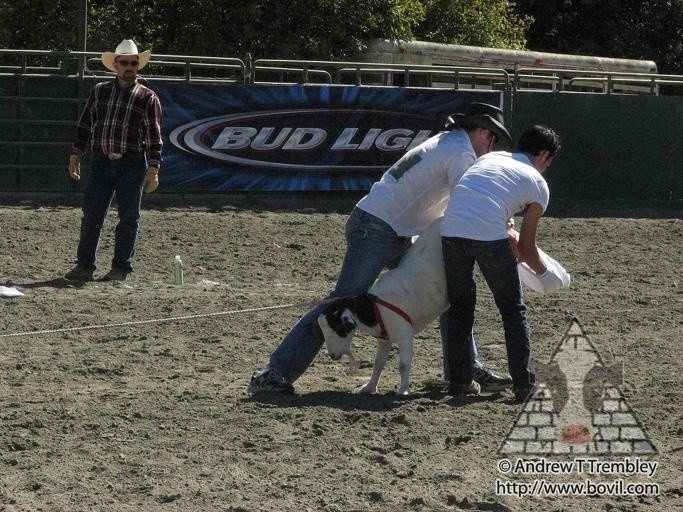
[95,152,143,160]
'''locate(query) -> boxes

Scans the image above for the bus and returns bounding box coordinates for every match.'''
[337,36,660,98]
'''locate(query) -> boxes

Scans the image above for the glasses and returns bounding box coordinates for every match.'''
[118,60,140,66]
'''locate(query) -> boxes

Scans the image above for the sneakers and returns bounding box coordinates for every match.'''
[64,266,93,281]
[447,380,481,396]
[473,368,512,392]
[249,371,295,394]
[103,267,127,281]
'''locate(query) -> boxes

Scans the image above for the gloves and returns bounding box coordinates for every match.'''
[68,154,82,181]
[143,166,159,193]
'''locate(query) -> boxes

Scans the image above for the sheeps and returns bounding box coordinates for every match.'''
[507,218,515,228]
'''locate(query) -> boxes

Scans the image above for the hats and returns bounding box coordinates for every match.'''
[444,102,513,145]
[101,39,151,72]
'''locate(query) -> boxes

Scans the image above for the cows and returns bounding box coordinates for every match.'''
[317,216,451,398]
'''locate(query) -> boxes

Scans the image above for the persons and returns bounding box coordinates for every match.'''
[64,39,164,282]
[436,122,559,399]
[248,101,514,396]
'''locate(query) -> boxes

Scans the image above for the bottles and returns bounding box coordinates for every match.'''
[173,255,184,286]
